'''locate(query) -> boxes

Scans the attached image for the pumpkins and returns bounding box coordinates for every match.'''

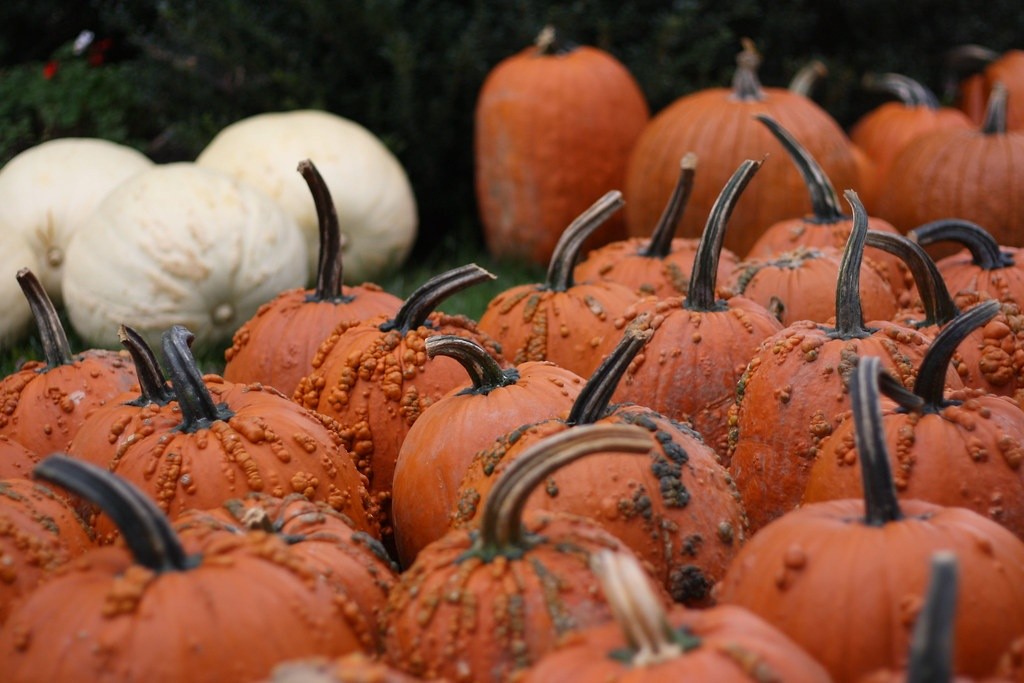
[0,27,1024,683]
